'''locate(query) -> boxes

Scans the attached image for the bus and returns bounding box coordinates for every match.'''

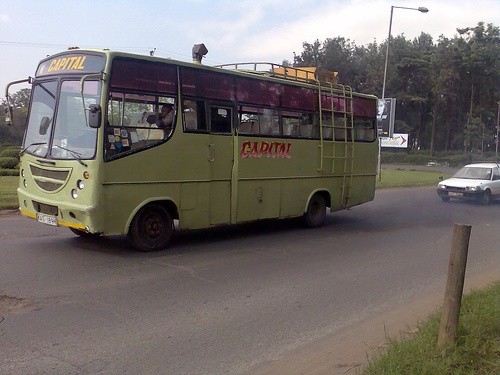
[5,43,381,252]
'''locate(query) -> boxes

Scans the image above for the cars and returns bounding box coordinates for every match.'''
[436,162,500,206]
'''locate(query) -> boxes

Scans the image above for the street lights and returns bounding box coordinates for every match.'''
[382,5,430,101]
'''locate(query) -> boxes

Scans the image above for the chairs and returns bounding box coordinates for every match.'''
[237,117,375,142]
